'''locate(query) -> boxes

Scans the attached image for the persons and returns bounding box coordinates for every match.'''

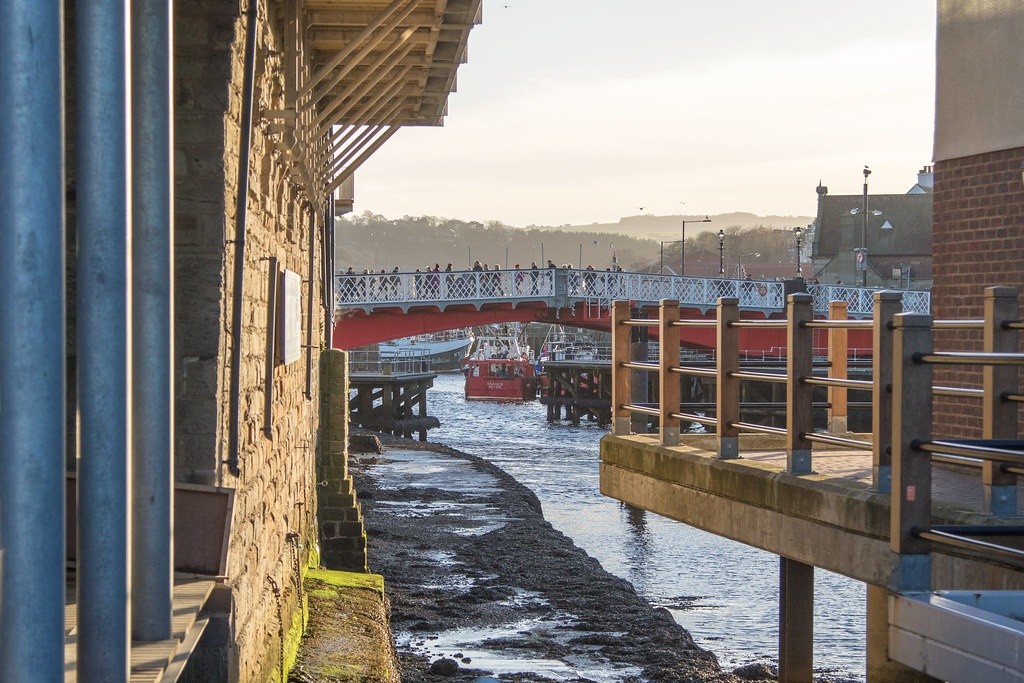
[338,260,624,302]
[743,275,847,305]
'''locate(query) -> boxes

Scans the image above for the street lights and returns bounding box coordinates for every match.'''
[661,240,687,274]
[682,219,711,275]
[795,227,803,277]
[718,229,725,278]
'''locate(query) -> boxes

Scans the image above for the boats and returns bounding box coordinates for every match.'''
[377,326,477,373]
[534,323,604,407]
[460,321,537,404]
